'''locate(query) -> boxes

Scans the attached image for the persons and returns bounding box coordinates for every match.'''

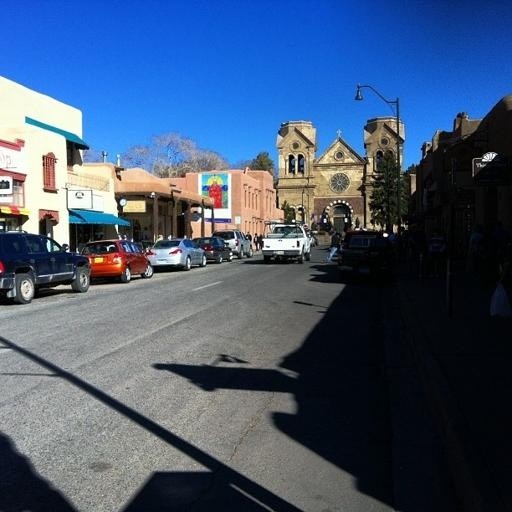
[323,229,344,264]
[246,232,265,251]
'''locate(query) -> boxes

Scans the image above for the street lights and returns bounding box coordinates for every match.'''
[353,83,401,283]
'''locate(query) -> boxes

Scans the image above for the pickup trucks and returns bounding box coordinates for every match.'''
[258,223,320,267]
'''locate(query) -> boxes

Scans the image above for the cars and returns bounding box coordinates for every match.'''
[307,230,319,247]
[332,232,387,270]
[0,227,257,305]
